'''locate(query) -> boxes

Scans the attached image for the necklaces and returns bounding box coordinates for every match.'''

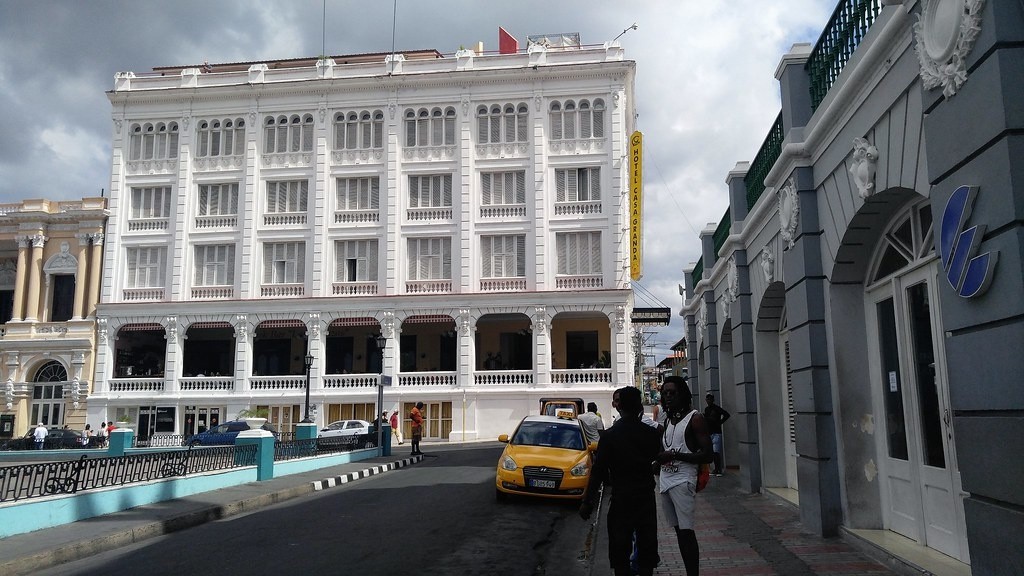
[665,417,677,448]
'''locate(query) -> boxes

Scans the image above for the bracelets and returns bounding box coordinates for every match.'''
[671,448,676,462]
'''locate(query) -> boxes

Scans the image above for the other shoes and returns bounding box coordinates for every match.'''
[709,469,722,477]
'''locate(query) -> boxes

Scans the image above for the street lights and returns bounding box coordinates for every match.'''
[300,350,315,423]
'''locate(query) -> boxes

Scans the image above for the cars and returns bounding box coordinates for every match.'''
[3,428,104,451]
[184,417,278,446]
[317,420,373,438]
[495,411,599,505]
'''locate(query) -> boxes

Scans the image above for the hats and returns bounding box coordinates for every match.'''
[706,392,714,398]
[393,409,399,413]
[382,410,388,413]
[38,423,43,425]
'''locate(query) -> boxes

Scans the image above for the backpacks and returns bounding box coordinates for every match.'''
[696,449,710,491]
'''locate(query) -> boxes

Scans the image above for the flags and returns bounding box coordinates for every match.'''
[498,26,517,54]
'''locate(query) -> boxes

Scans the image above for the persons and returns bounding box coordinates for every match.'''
[81,424,92,449]
[98,422,117,448]
[483,350,502,370]
[651,376,713,576]
[578,386,665,576]
[703,393,729,477]
[389,410,404,444]
[372,410,389,431]
[34,423,48,450]
[652,391,669,426]
[409,402,425,455]
